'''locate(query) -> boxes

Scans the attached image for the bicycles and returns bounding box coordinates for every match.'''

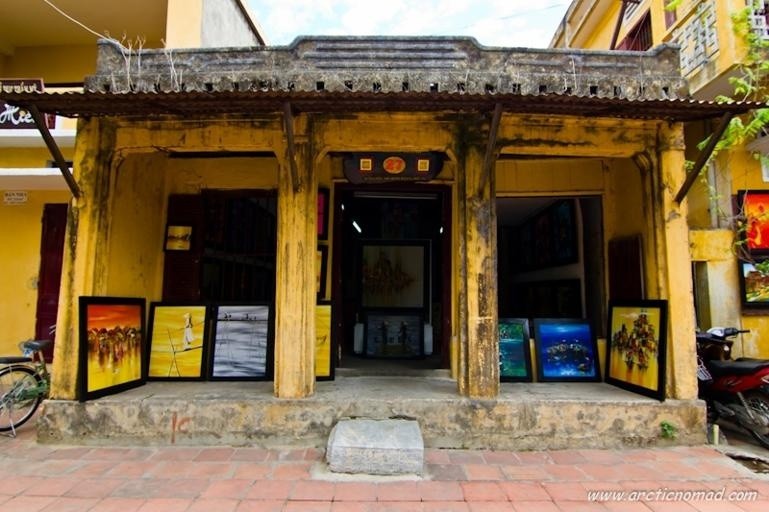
[0,324,57,439]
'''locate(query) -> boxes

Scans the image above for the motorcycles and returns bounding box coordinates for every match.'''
[696,326,769,451]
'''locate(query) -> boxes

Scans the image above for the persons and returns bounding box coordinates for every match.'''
[182,311,196,352]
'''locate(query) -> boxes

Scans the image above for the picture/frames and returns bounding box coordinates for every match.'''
[738,190,769,254]
[604,300,668,401]
[358,239,430,315]
[534,318,602,383]
[315,299,336,381]
[738,257,769,316]
[146,300,214,381]
[211,302,274,381]
[79,295,147,402]
[498,318,532,382]
